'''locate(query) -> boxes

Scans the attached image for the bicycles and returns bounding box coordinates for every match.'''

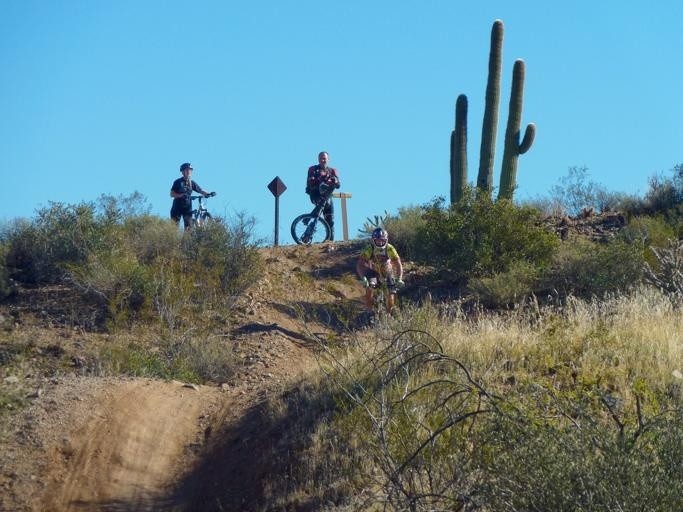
[366,281,401,327]
[289,176,340,245]
[184,191,217,231]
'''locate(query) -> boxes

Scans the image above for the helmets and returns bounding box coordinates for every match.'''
[372,228,388,249]
[179,162,192,171]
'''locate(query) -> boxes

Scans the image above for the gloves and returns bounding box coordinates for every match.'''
[395,279,405,287]
[360,276,369,287]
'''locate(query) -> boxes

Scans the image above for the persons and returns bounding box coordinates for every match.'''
[307,152,341,241]
[357,228,403,319]
[170,163,215,232]
